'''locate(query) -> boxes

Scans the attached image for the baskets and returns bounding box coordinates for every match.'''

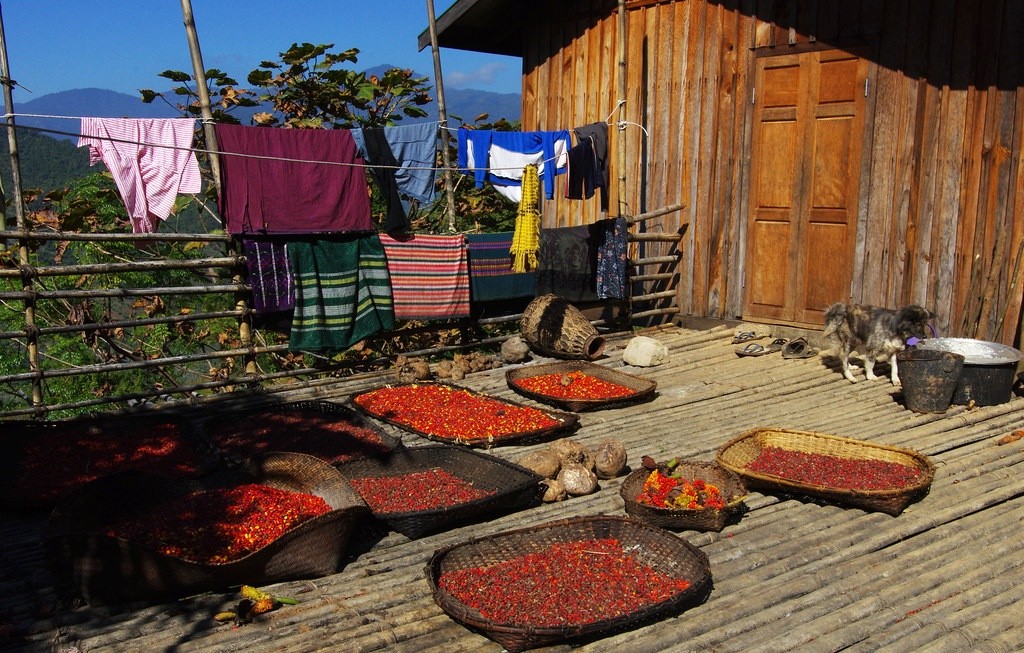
[1,359,935,653]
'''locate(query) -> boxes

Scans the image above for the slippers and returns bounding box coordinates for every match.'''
[731,330,819,359]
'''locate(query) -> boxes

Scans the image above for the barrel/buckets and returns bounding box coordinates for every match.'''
[896,350,964,414]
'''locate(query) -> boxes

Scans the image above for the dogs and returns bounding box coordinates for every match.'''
[821,303,939,386]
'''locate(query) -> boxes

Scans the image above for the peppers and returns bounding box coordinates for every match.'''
[12,371,923,626]
